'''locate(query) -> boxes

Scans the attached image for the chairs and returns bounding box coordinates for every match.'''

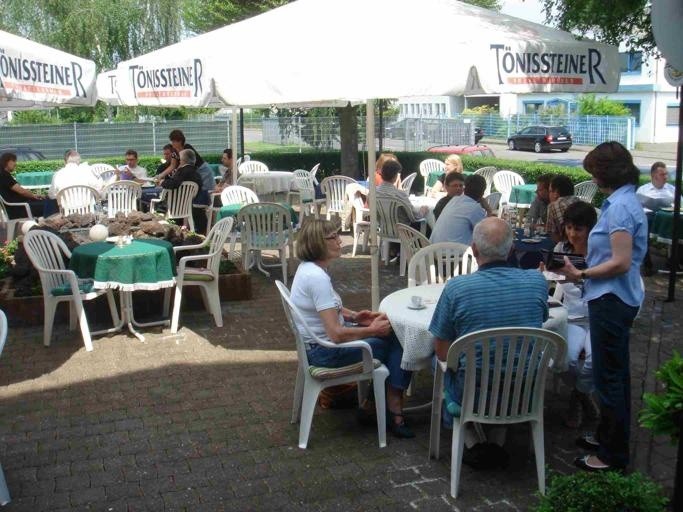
[23,230,95,351]
[345,183,371,249]
[321,175,358,232]
[0,194,33,221]
[288,165,318,205]
[575,180,600,200]
[105,181,142,221]
[239,161,269,174]
[419,159,448,175]
[89,164,119,180]
[493,170,525,219]
[481,193,503,213]
[395,224,431,276]
[429,327,569,499]
[274,280,390,449]
[237,203,292,286]
[402,173,417,194]
[0,310,11,506]
[150,181,199,233]
[57,185,101,217]
[382,205,426,267]
[219,186,259,206]
[408,241,487,288]
[163,217,234,333]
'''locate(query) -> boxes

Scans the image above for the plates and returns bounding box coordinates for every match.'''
[105,235,133,243]
[407,303,426,310]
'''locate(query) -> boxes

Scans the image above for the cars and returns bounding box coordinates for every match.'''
[427,122,484,144]
[384,118,428,140]
[507,125,572,153]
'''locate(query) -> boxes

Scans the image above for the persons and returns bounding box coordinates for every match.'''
[366,160,429,262]
[140,149,203,215]
[525,172,560,238]
[114,150,147,183]
[155,144,176,179]
[48,149,102,213]
[215,149,233,192]
[545,173,585,245]
[364,153,402,220]
[288,220,416,439]
[428,176,495,248]
[428,216,548,473]
[432,172,464,221]
[152,128,217,211]
[635,159,683,212]
[558,141,649,472]
[550,200,603,431]
[431,152,469,199]
[0,154,59,219]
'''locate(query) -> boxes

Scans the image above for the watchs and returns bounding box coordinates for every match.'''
[581,269,586,280]
[350,311,355,323]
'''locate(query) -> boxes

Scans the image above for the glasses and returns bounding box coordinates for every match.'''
[324,233,338,241]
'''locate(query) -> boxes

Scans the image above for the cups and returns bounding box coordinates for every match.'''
[412,294,422,307]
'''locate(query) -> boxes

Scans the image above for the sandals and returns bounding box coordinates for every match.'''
[576,433,601,450]
[355,405,380,420]
[568,457,625,475]
[381,411,414,439]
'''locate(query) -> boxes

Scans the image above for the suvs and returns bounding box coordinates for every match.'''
[0,144,47,161]
[425,144,496,157]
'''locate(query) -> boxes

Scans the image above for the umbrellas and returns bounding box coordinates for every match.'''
[1,28,96,116]
[96,0,619,312]
